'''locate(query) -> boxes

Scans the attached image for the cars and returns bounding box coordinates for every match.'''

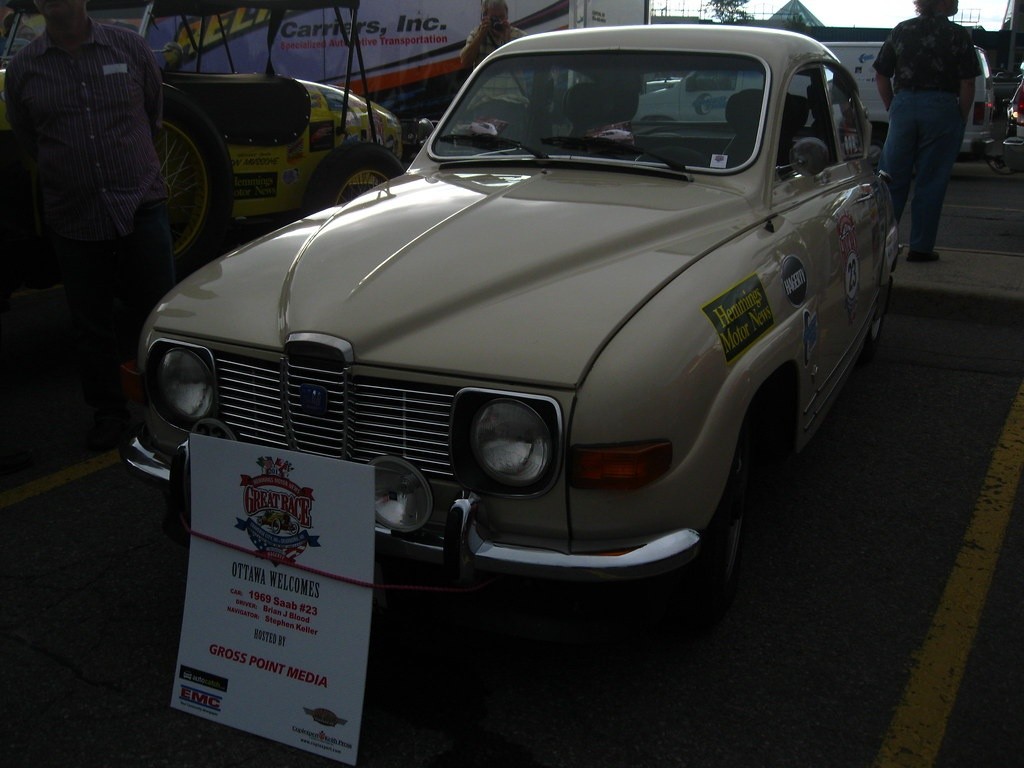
[0,0,404,300]
[1001,76,1024,173]
[117,23,903,624]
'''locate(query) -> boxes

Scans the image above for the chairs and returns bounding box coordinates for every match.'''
[721,88,801,173]
[554,82,640,156]
[789,94,817,137]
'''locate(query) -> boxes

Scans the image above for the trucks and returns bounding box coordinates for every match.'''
[630,41,994,163]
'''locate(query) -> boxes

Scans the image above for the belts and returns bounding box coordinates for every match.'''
[897,86,959,98]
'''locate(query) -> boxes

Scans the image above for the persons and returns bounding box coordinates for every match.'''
[872,0,983,263]
[5,0,176,454]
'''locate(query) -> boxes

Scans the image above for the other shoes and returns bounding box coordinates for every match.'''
[905,250,940,263]
[86,410,132,451]
